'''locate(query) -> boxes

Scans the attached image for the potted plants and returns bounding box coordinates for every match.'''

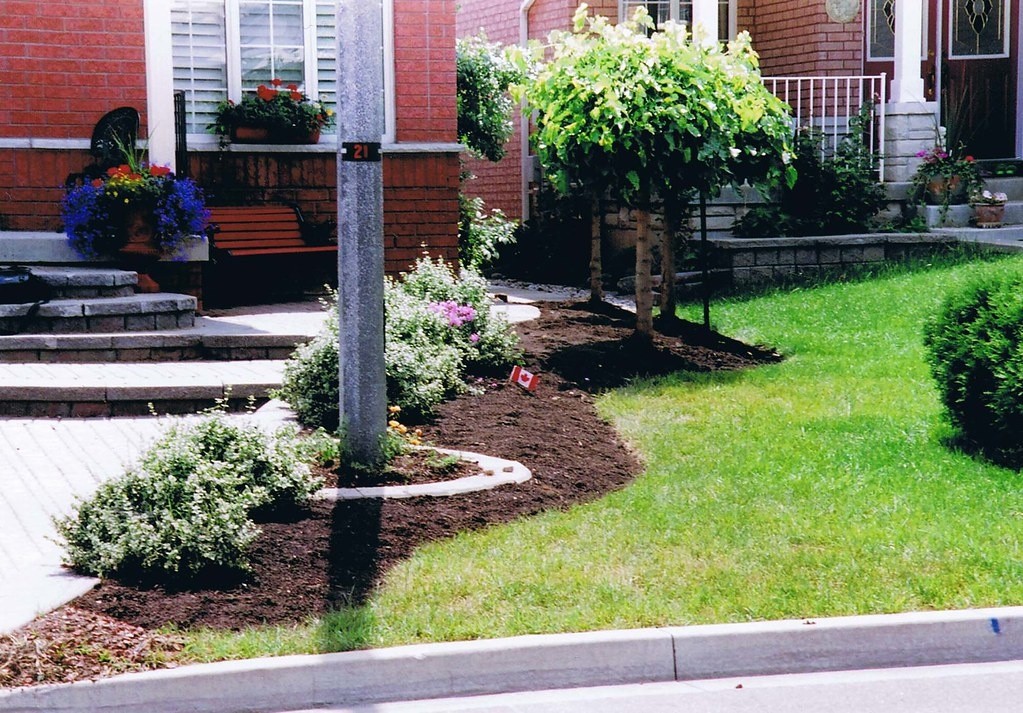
[738,105,894,233]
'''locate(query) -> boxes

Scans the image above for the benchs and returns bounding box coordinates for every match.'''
[202,205,339,297]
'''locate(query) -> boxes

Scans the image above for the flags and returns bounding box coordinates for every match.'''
[510,365,539,390]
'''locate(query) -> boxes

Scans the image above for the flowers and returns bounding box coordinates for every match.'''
[214,80,334,133]
[64,159,209,265]
[902,146,1007,227]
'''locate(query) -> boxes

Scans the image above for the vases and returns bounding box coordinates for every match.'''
[273,121,320,145]
[227,125,269,145]
[157,230,212,262]
[971,201,1006,228]
[923,171,966,205]
